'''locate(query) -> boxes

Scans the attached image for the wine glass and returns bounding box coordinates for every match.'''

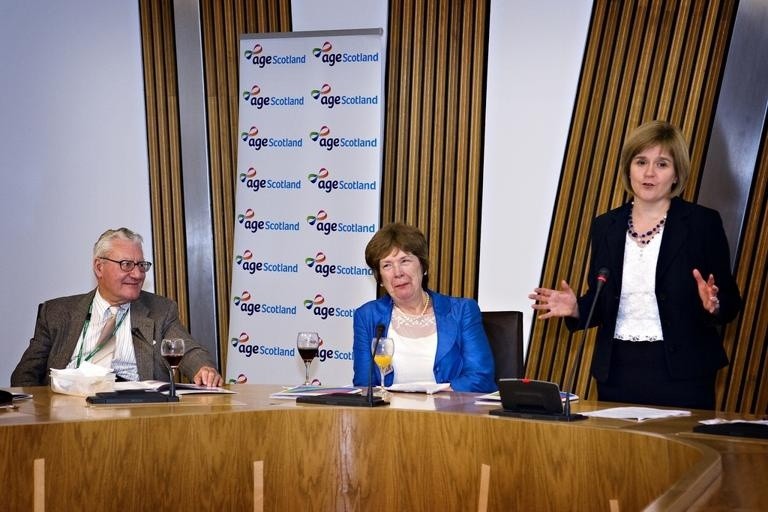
[370,336,395,398]
[160,337,185,401]
[296,331,320,385]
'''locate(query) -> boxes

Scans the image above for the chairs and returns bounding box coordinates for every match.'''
[480,309,522,389]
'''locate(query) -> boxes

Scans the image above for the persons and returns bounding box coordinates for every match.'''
[23,385,222,422]
[356,386,487,415]
[352,222,500,396]
[529,119,746,411]
[595,401,719,438]
[9,227,226,389]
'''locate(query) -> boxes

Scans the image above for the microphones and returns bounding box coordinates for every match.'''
[365,321,386,406]
[129,324,180,403]
[561,264,611,423]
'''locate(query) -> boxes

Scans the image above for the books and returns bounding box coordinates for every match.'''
[267,384,363,400]
[474,389,579,403]
[113,379,238,397]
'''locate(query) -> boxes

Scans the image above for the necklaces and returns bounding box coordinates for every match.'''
[627,210,669,237]
[628,202,662,245]
[393,291,431,321]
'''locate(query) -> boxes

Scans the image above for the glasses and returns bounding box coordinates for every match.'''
[99,257,152,274]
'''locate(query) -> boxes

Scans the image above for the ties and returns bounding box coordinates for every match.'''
[91,306,121,369]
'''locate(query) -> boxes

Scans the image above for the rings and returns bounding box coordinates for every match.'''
[712,299,719,304]
